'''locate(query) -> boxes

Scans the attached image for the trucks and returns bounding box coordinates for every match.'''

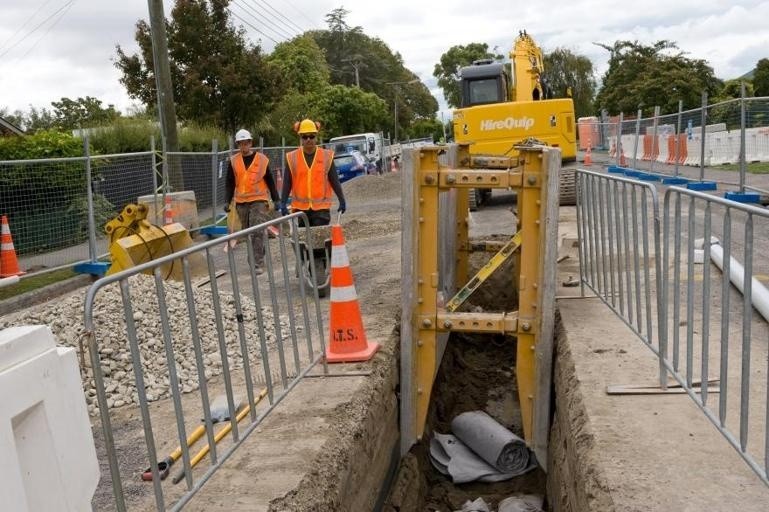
[329,131,402,175]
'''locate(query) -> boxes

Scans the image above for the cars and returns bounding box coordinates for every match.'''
[333,149,381,183]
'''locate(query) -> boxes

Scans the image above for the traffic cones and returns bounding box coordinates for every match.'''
[611,139,628,167]
[165,193,174,224]
[0,216,27,278]
[276,169,283,192]
[324,223,379,363]
[390,158,398,172]
[583,137,593,166]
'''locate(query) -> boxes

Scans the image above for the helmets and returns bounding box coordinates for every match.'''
[294,119,321,134]
[235,129,252,142]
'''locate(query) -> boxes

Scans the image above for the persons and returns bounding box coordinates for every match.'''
[280,118,347,280]
[223,127,280,276]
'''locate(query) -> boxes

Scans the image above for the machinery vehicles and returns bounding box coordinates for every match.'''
[453,30,583,211]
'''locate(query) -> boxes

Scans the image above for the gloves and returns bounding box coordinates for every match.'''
[224,202,230,212]
[274,202,289,215]
[337,201,345,213]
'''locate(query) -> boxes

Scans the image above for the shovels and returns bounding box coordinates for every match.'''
[142,394,242,479]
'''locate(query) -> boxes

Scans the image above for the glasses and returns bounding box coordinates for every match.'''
[301,135,314,139]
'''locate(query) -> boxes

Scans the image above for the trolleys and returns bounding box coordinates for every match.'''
[285,210,342,298]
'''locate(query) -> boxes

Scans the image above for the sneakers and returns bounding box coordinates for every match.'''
[255,266,263,274]
